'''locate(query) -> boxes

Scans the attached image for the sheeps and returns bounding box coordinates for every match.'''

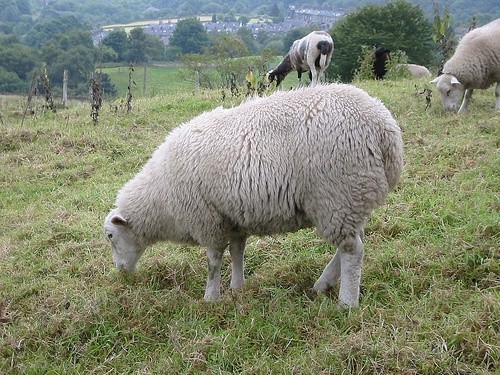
[103,77,405,310]
[428,17,500,114]
[373,46,431,80]
